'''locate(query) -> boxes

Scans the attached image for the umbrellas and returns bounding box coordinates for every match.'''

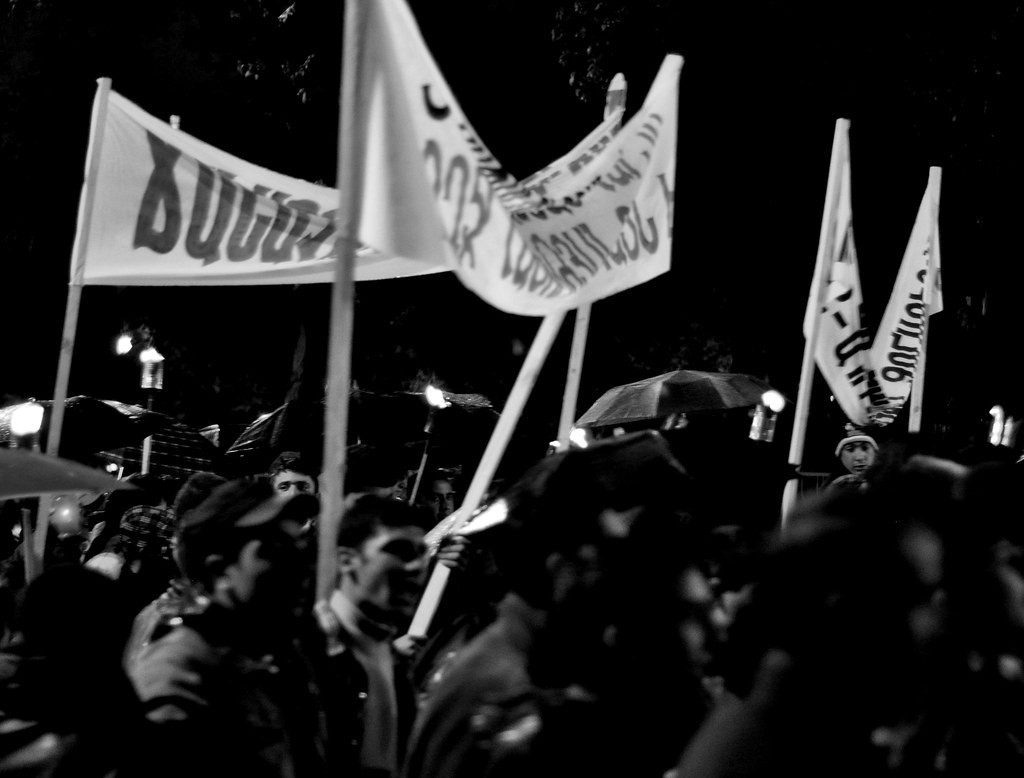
[575,368,786,432]
[0,383,502,503]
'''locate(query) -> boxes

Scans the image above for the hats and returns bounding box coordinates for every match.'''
[173,476,319,560]
[835,422,880,457]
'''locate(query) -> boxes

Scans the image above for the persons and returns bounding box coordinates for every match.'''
[0,425,1023,778]
